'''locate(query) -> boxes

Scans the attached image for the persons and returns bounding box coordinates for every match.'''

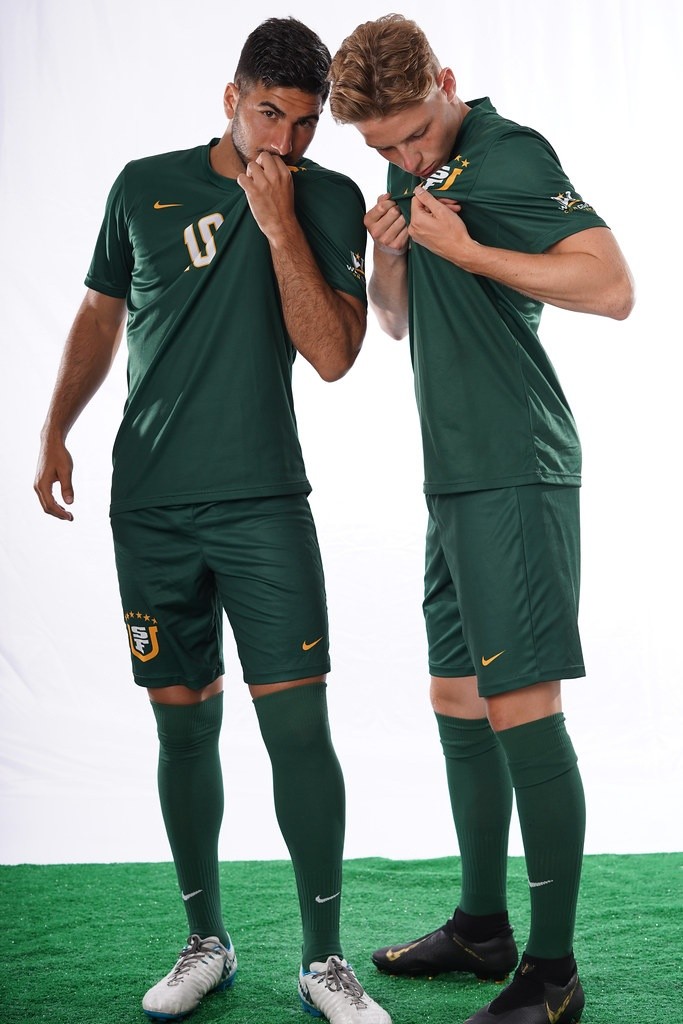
[326,12,636,1023]
[32,19,394,1024]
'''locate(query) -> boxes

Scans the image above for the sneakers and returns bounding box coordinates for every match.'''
[459,951,585,1024]
[297,955,392,1024]
[371,920,518,984]
[142,931,238,1020]
